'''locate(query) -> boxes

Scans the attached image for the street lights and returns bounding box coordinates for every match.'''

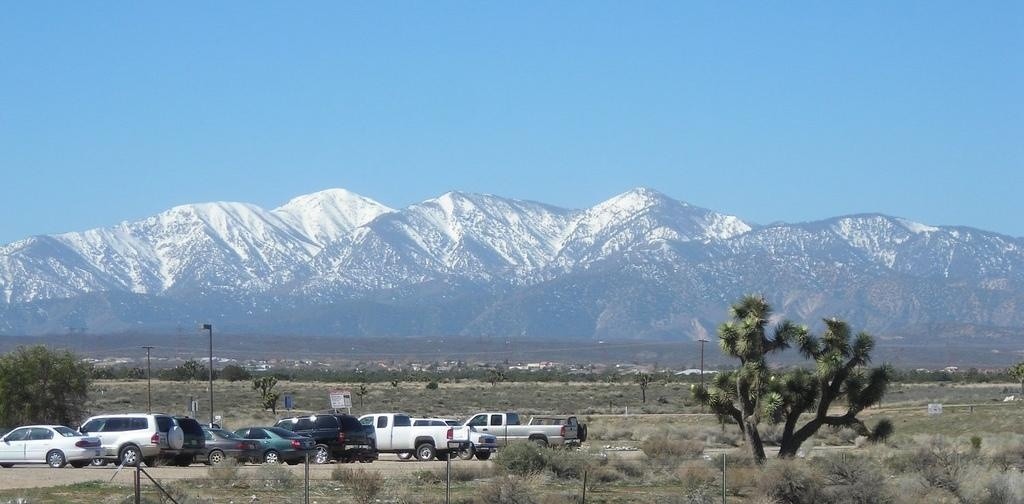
[143,343,157,412]
[201,323,214,426]
[695,336,711,414]
